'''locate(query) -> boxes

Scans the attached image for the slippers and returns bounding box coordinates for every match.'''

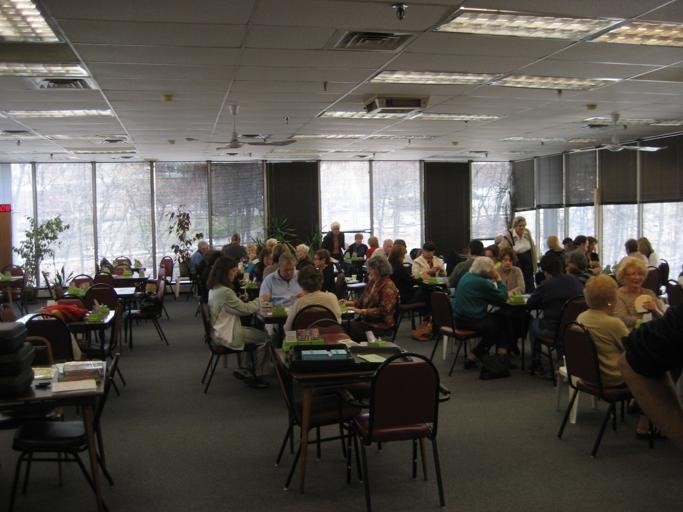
[635,425,665,440]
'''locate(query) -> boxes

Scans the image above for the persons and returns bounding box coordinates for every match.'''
[321,223,345,265]
[189,222,312,300]
[258,253,305,376]
[447,217,601,373]
[342,233,448,318]
[315,249,336,294]
[573,237,682,456]
[206,256,269,388]
[273,264,342,352]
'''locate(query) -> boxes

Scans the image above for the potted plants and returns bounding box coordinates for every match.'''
[166,204,204,278]
[11,212,70,301]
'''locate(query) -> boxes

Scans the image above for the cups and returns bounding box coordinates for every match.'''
[352,274,357,281]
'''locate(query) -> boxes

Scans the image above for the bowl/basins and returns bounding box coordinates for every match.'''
[510,295,525,303]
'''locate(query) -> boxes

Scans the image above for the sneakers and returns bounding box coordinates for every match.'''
[464,358,480,369]
[247,377,268,389]
[234,369,254,380]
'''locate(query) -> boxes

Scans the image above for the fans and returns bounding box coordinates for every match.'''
[569,115,668,154]
[206,104,296,150]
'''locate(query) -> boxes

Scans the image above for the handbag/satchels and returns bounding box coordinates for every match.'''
[412,322,432,342]
[140,292,161,319]
[41,305,88,322]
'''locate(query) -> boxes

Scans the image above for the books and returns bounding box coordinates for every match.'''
[30,361,104,395]
